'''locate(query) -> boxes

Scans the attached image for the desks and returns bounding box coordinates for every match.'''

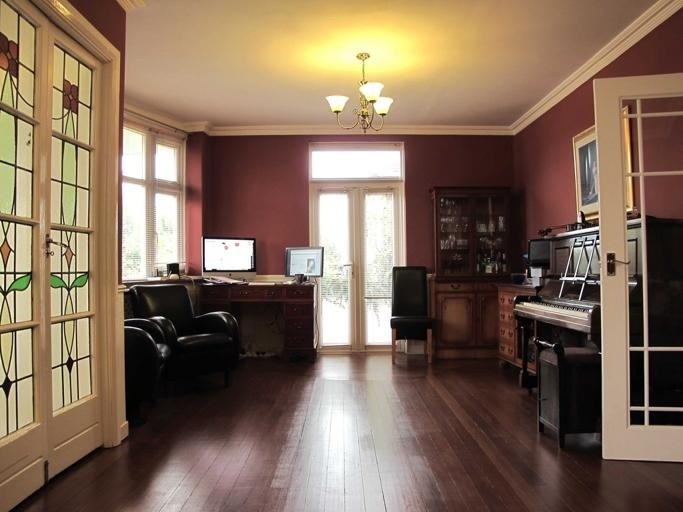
[200,279,317,364]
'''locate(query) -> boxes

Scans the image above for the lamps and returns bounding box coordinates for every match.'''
[326,51,394,134]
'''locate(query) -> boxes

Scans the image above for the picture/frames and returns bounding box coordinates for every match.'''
[572,105,635,224]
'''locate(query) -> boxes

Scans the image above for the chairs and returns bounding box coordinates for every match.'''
[390,265,434,366]
[533,336,601,449]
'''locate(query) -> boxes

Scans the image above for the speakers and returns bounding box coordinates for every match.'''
[167,263,179,276]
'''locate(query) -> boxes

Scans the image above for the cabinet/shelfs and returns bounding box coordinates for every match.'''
[429,183,525,276]
[498,284,537,383]
[430,271,499,359]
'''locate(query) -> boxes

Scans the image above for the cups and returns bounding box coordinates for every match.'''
[510,274,525,285]
[440,235,469,250]
[441,197,459,215]
[295,273,304,286]
[439,217,469,234]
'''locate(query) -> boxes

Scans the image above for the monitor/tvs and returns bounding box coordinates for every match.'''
[200,236,257,281]
[285,246,326,281]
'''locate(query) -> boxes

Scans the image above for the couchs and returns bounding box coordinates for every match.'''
[118,318,169,429]
[127,281,239,389]
